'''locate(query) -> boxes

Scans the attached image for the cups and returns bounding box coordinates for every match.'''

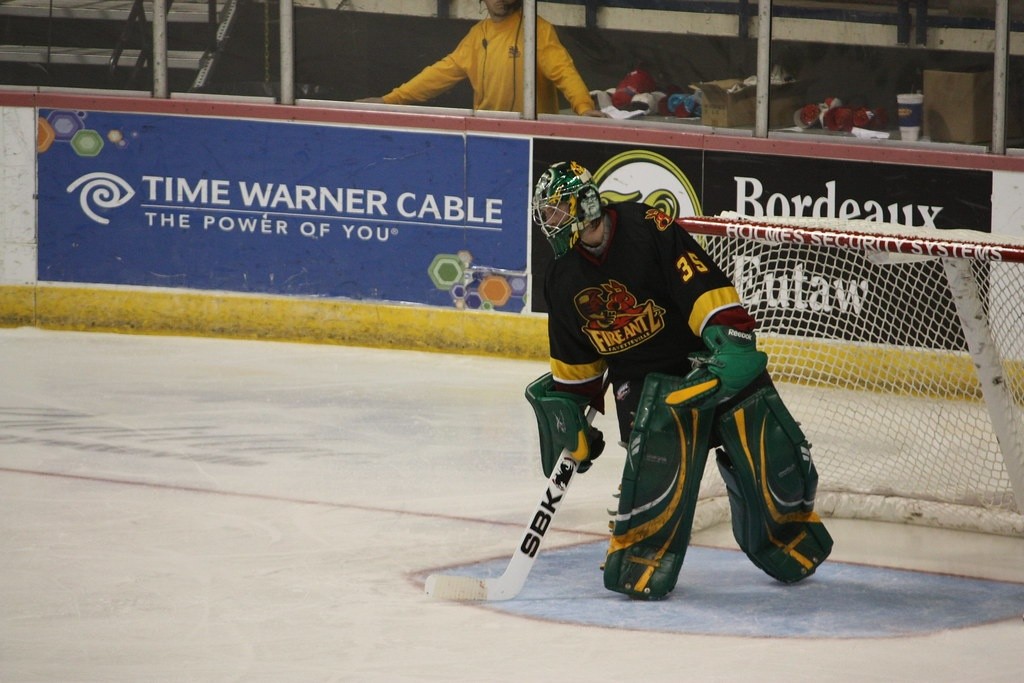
[896,94,924,140]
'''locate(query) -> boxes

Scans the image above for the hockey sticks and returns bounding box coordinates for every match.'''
[423,363,612,602]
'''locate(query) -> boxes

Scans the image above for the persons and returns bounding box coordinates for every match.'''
[355,0,606,120]
[522,161,833,601]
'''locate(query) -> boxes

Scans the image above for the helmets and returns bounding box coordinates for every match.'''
[532,161,601,260]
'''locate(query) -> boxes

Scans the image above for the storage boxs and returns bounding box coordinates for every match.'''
[689,79,752,128]
[922,67,1024,145]
[754,78,816,130]
